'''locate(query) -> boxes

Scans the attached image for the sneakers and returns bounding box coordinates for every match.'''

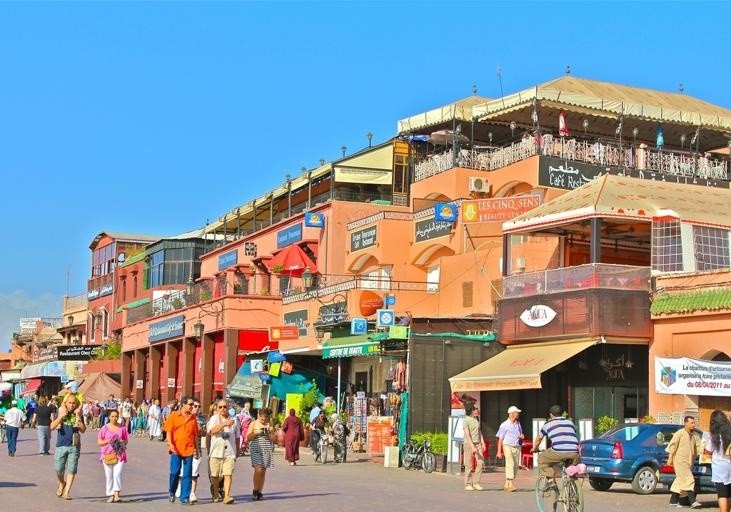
[473,484,482,490]
[57,482,72,499]
[503,484,518,492]
[170,485,198,504]
[291,462,297,465]
[691,501,702,508]
[252,489,266,500]
[106,496,121,503]
[543,479,557,492]
[465,485,474,490]
[9,451,51,457]
[670,503,683,508]
[210,485,234,503]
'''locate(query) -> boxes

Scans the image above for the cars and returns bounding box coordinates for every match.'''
[661,452,718,495]
[578,423,717,495]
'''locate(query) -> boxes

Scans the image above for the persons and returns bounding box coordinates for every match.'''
[666,417,703,507]
[176,398,207,502]
[1,393,179,466]
[705,410,731,512]
[166,395,200,503]
[463,402,487,491]
[530,406,578,491]
[50,395,86,501]
[59,381,83,430]
[97,411,128,505]
[206,396,346,505]
[497,406,524,492]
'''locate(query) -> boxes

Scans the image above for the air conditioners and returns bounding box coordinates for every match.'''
[469,177,489,194]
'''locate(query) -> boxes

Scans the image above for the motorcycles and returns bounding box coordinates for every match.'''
[401,440,436,473]
[312,428,334,464]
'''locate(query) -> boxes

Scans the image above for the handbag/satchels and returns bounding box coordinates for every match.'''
[328,435,334,444]
[105,454,117,464]
[72,432,80,445]
[342,425,350,435]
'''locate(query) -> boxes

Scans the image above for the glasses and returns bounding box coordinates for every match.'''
[187,403,227,409]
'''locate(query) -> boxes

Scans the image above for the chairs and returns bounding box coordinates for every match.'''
[460,439,534,471]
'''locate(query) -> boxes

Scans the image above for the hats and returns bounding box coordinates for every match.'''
[12,401,17,404]
[508,406,521,413]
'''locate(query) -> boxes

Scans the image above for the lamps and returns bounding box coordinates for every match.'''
[301,266,347,314]
[68,315,74,326]
[194,300,223,342]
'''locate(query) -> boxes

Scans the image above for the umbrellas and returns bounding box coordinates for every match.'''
[267,244,319,298]
[657,122,664,150]
[559,109,568,148]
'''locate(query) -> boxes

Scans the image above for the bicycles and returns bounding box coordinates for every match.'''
[532,449,586,512]
